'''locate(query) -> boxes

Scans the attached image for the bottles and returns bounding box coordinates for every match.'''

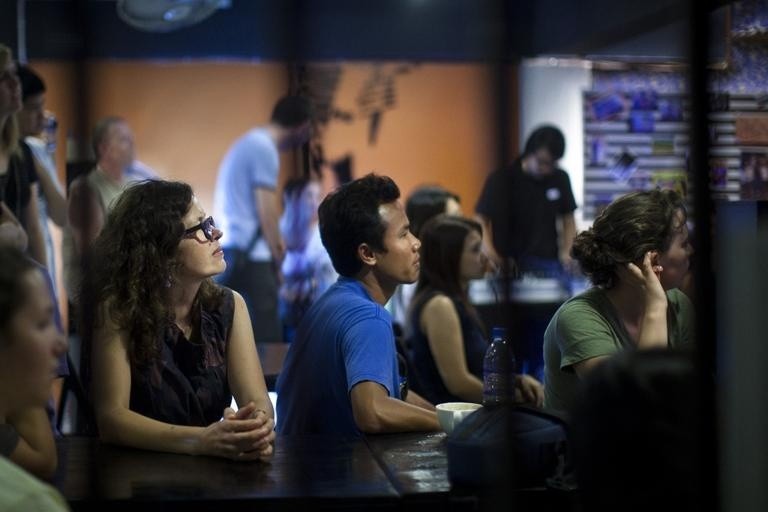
[482,327,516,406]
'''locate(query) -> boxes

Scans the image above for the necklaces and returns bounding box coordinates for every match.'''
[182,324,190,334]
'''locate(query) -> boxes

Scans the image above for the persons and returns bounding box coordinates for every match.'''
[473,124,577,388]
[214,89,340,342]
[564,342,717,511]
[1,46,70,510]
[381,186,546,409]
[543,187,693,410]
[275,170,442,444]
[69,176,275,462]
[69,115,136,290]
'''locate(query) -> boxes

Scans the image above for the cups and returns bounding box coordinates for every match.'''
[434,400,482,437]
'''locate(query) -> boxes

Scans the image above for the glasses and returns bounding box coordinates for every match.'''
[179,215,215,241]
